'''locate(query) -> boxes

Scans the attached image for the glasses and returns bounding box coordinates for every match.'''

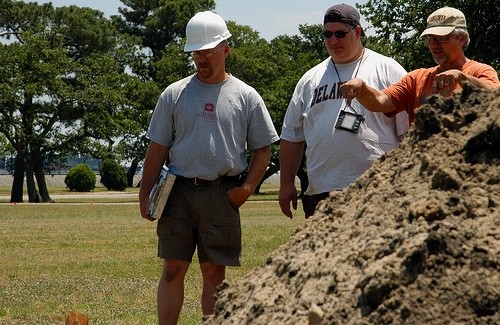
[322,28,353,38]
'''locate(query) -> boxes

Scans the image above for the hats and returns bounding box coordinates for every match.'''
[420,6,466,38]
[324,3,366,37]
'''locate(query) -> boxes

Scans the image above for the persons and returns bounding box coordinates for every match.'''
[341,6,500,124]
[280,3,410,219]
[139,11,280,325]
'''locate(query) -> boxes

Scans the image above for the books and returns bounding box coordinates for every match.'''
[146,165,176,220]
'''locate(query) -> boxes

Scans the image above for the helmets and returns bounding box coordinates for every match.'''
[184,10,232,52]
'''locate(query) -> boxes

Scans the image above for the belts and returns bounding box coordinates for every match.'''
[176,175,235,185]
